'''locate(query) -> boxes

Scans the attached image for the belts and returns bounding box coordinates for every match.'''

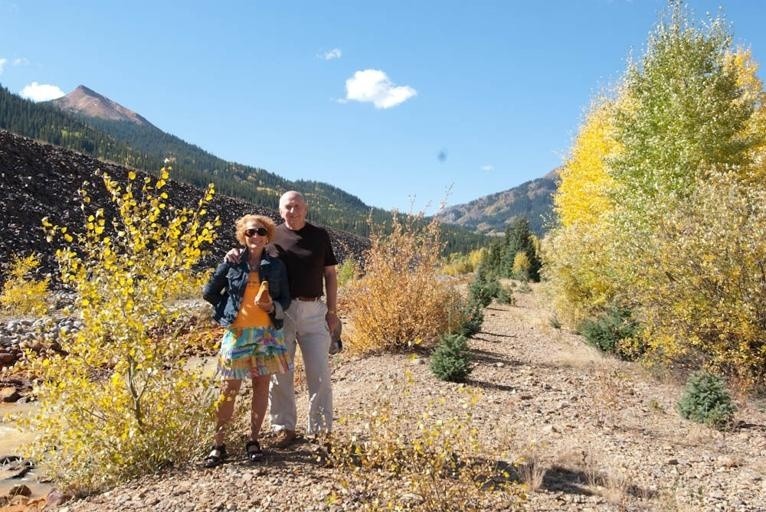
[294,296,321,301]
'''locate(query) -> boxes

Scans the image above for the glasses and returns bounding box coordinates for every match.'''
[245,228,268,237]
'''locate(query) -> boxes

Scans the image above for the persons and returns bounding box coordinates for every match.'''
[225,189,341,469]
[202,212,292,469]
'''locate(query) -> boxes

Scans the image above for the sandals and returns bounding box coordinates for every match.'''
[246,441,264,461]
[202,444,225,466]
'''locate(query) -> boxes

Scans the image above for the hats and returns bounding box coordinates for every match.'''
[326,314,342,354]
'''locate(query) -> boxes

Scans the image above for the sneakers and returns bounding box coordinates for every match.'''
[272,428,296,448]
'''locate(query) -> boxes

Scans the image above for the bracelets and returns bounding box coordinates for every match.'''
[328,309,337,314]
[267,305,275,314]
